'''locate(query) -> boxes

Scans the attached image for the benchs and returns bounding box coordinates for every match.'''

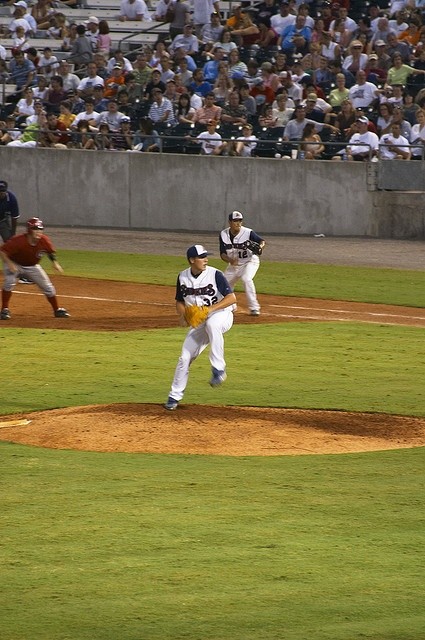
[0,0,242,108]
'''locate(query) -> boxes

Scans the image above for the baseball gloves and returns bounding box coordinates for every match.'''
[242,241,263,256]
[182,306,209,328]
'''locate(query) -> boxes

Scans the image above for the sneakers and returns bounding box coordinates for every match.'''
[251,310,261,315]
[211,369,227,384]
[164,397,178,411]
[54,308,70,318]
[0,307,11,320]
[18,276,33,284]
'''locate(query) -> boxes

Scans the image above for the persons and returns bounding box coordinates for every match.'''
[1,216,71,319]
[219,210,265,316]
[0,1,425,163]
[163,244,237,411]
[1,180,33,284]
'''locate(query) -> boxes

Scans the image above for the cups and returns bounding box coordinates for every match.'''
[291,150,299,159]
[274,152,281,157]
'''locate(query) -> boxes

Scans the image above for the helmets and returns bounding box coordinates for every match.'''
[28,217,44,230]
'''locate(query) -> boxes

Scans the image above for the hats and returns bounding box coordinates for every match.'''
[13,1,26,9]
[259,62,272,70]
[187,245,213,258]
[152,87,162,94]
[356,115,370,125]
[34,99,42,105]
[113,63,122,69]
[233,4,241,10]
[254,77,264,86]
[279,71,291,81]
[152,68,162,74]
[243,123,253,130]
[322,1,331,8]
[289,36,303,43]
[211,11,220,17]
[0,181,8,192]
[307,93,318,102]
[369,54,379,62]
[94,84,103,90]
[229,211,244,221]
[206,121,217,126]
[167,79,175,84]
[298,76,312,84]
[230,72,243,80]
[375,39,387,47]
[83,16,98,25]
[120,116,131,122]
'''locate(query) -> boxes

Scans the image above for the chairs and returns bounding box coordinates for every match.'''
[158,129,348,159]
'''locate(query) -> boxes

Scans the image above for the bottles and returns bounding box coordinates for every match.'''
[342,150,348,161]
